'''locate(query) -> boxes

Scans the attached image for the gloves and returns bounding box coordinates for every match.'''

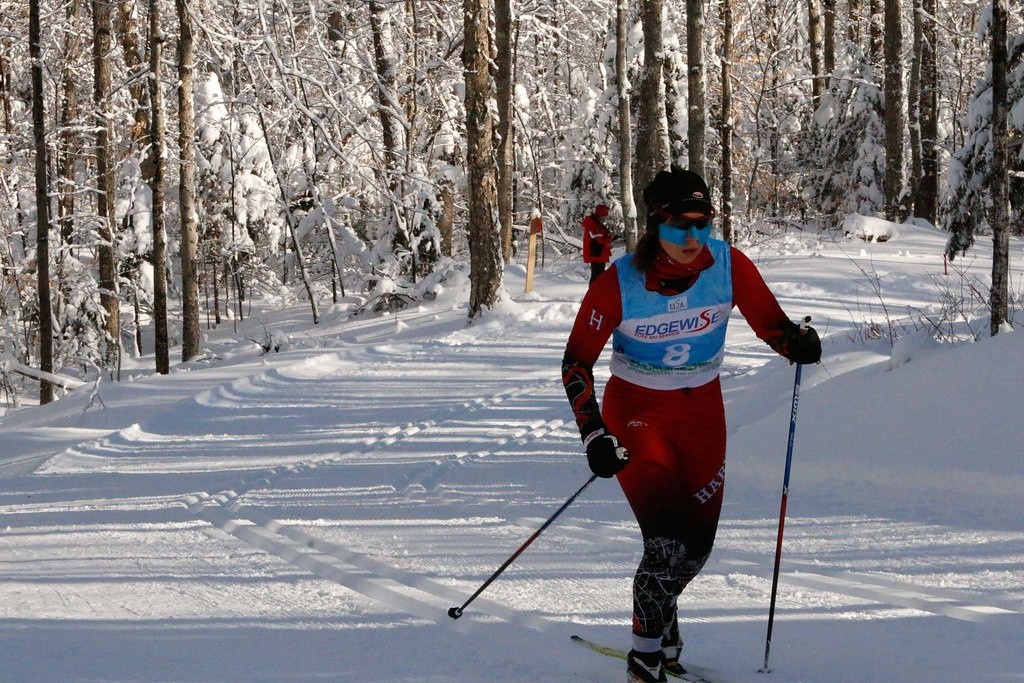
[777,324,822,364]
[581,417,630,478]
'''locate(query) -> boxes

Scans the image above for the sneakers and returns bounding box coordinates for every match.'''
[662,645,689,675]
[626,647,668,683]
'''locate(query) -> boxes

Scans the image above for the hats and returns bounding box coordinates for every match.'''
[595,205,609,217]
[643,164,715,226]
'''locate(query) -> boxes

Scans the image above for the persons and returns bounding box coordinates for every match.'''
[562,164,822,683]
[581,205,614,287]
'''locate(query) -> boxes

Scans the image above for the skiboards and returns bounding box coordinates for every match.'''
[568,633,710,683]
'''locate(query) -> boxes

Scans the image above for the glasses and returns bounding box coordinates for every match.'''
[656,214,711,231]
